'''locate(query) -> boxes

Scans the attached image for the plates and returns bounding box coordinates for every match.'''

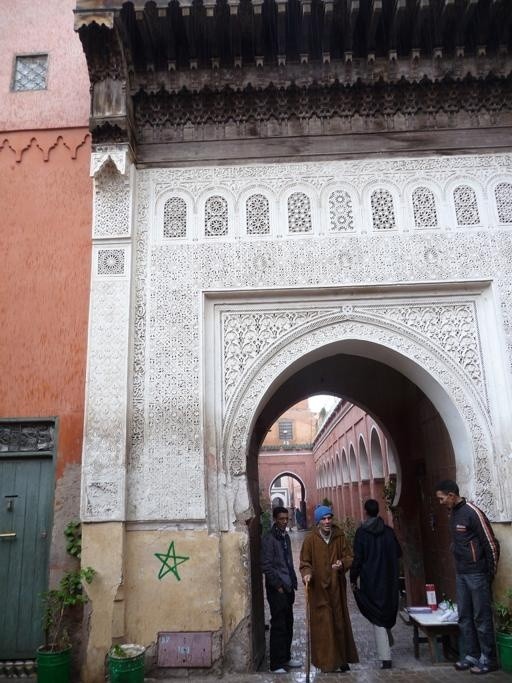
[405,606,432,612]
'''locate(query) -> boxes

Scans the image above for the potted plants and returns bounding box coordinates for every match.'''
[35,519,95,682]
[492,586,511,675]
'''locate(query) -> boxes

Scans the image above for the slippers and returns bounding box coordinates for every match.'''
[453,658,479,671]
[470,662,500,675]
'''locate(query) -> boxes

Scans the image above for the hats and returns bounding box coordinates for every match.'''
[313,505,334,526]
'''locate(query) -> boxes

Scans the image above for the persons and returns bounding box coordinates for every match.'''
[296,508,303,531]
[434,478,500,675]
[350,499,402,669]
[262,505,303,675]
[299,505,359,672]
[288,507,295,532]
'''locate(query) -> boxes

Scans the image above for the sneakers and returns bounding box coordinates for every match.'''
[380,660,392,669]
[269,668,288,674]
[282,659,303,667]
[340,663,350,672]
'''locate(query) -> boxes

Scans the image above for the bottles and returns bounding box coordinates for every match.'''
[425,583,437,609]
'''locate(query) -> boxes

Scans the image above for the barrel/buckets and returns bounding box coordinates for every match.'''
[107,643,146,683]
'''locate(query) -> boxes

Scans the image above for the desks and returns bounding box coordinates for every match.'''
[401,604,460,663]
